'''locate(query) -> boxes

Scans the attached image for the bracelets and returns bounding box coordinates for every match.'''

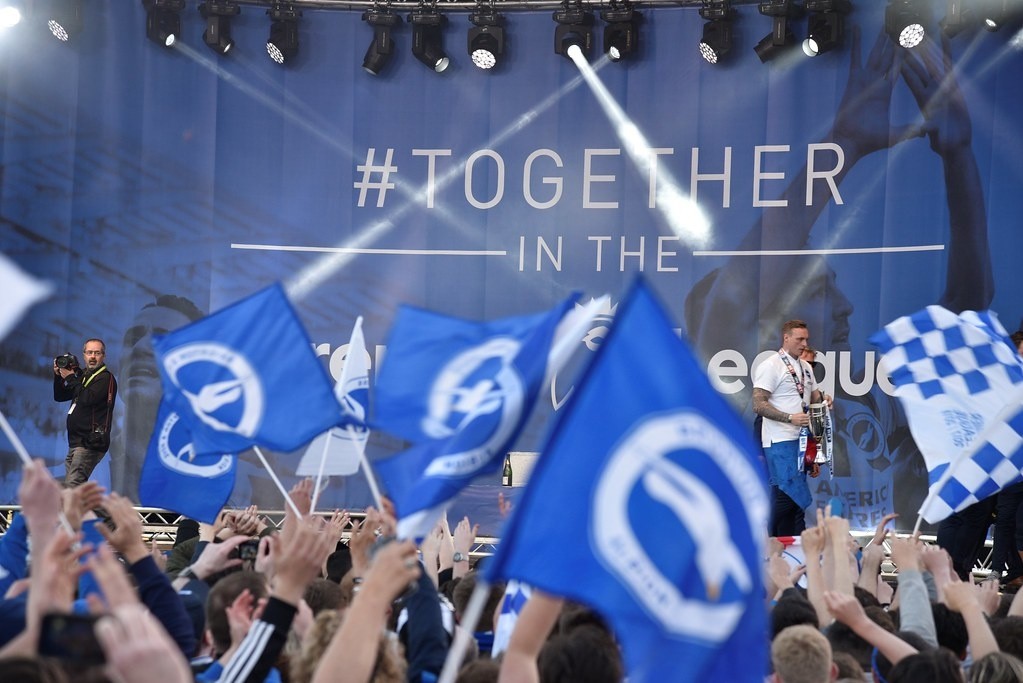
[351,577,361,594]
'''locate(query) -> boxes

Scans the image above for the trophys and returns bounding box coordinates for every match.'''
[804,403,828,463]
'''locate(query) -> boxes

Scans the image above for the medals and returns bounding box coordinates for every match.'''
[801,401,806,407]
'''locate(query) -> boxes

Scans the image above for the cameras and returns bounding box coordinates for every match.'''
[55,354,80,373]
[238,540,270,560]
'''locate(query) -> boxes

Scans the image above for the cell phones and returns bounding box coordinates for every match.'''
[371,536,419,600]
[35,613,105,664]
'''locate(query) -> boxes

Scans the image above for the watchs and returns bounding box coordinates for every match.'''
[452,552,469,562]
[788,414,793,423]
[177,567,199,581]
[883,606,889,613]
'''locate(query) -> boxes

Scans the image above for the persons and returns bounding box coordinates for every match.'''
[0,459,627,683]
[109,293,206,507]
[53,340,117,490]
[762,496,1023,683]
[1011,331,1023,353]
[754,321,834,536]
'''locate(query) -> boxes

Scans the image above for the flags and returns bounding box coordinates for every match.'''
[490,579,531,657]
[470,276,770,683]
[866,306,1023,525]
[298,310,372,476]
[139,389,236,524]
[371,292,585,521]
[150,285,348,458]
